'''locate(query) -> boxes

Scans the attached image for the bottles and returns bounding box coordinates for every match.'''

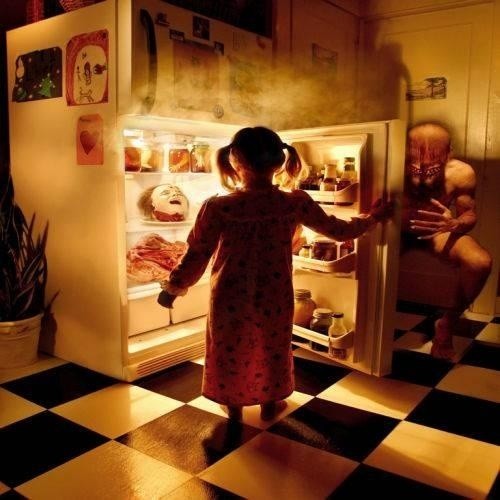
[169,142,190,173]
[190,141,213,173]
[320,163,340,191]
[298,234,338,273]
[124,135,163,172]
[6,1,408,388]
[298,166,318,191]
[334,156,358,206]
[291,288,317,343]
[310,307,334,352]
[329,312,348,359]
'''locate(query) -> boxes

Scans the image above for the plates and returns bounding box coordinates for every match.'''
[138,216,196,226]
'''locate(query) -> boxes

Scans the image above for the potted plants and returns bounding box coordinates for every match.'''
[0,174,49,370]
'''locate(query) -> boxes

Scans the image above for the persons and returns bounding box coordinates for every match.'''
[400,122,493,362]
[153,126,399,446]
